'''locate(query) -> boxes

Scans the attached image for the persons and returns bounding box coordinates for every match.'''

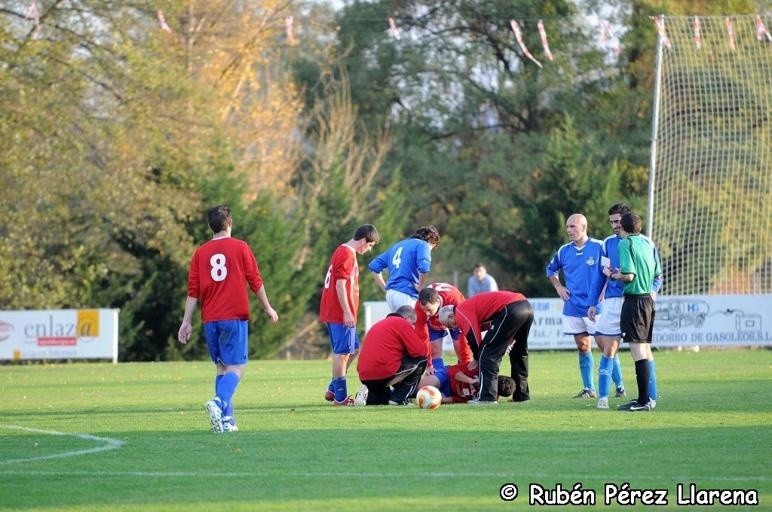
[353,202,665,413]
[175,204,281,435]
[318,223,381,408]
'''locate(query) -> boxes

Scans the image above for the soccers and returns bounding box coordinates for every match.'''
[416,385,442,408]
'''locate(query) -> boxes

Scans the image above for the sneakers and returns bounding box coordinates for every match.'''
[325,385,412,405]
[573,386,656,411]
[205,401,224,434]
[222,418,238,432]
[467,399,497,403]
